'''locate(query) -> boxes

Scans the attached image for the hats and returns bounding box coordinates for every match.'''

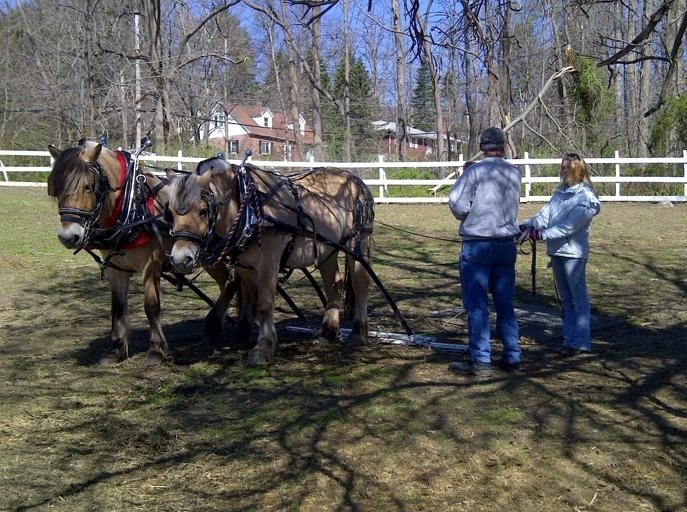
[478,127,504,146]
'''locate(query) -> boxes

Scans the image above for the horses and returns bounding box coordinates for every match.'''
[164,156,375,367]
[46,134,242,360]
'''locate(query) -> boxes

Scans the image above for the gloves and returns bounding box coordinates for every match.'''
[518,223,527,236]
[528,227,542,241]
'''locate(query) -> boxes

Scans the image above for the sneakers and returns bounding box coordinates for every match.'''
[552,345,585,355]
[503,359,519,373]
[449,360,491,377]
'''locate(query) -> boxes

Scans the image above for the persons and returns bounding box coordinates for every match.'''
[519,153,601,356]
[448,127,522,376]
